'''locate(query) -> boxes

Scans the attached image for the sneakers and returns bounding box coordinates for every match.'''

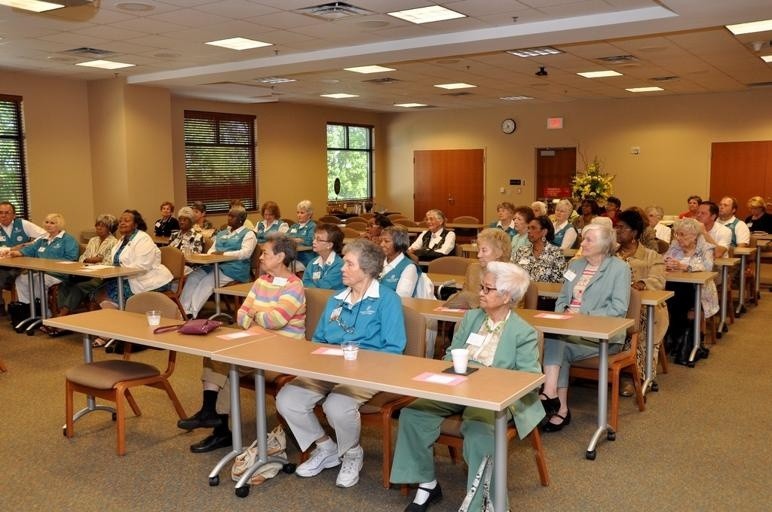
[296,447,342,478]
[336,448,364,488]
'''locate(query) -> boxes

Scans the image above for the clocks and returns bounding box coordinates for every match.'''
[501,119,516,134]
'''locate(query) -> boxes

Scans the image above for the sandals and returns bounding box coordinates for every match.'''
[92,337,106,348]
[40,324,73,338]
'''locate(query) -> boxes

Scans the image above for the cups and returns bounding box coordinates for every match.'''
[341,340,359,360]
[183,246,191,260]
[145,311,161,325]
[450,349,470,374]
[471,240,477,250]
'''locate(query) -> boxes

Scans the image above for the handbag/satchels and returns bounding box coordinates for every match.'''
[153,318,224,334]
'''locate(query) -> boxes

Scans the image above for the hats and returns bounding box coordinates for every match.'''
[176,206,196,224]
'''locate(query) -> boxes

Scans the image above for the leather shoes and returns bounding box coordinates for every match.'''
[538,391,571,433]
[190,430,232,453]
[177,410,222,429]
[404,481,442,512]
[620,376,635,397]
[665,330,686,357]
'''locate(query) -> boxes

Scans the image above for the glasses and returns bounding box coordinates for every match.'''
[311,233,333,245]
[611,226,635,233]
[337,304,359,335]
[477,283,498,294]
[365,223,381,229]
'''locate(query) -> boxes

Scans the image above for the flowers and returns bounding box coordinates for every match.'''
[561,150,617,206]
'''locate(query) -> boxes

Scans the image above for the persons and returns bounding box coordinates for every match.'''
[86,208,174,350]
[175,234,307,451]
[0,201,50,321]
[441,191,772,435]
[387,259,541,509]
[274,237,409,490]
[365,214,421,298]
[407,209,457,256]
[6,211,78,318]
[154,199,348,320]
[35,212,116,340]
